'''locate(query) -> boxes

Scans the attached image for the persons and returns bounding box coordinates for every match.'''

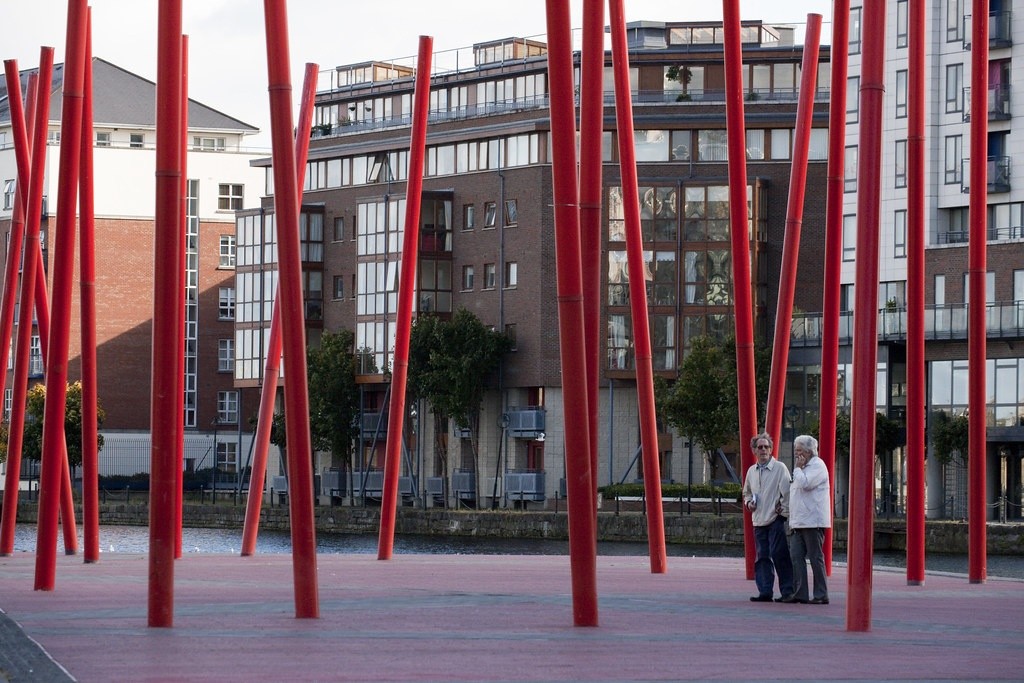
[742,432,792,602]
[783,435,831,604]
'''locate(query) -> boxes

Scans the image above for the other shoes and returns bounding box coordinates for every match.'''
[750,594,773,602]
[784,596,809,604]
[809,597,829,604]
[775,597,783,601]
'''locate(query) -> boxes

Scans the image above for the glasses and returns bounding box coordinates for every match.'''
[758,445,769,449]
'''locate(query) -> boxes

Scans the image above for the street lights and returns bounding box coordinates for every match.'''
[490,414,511,511]
[209,416,223,505]
[786,403,801,484]
